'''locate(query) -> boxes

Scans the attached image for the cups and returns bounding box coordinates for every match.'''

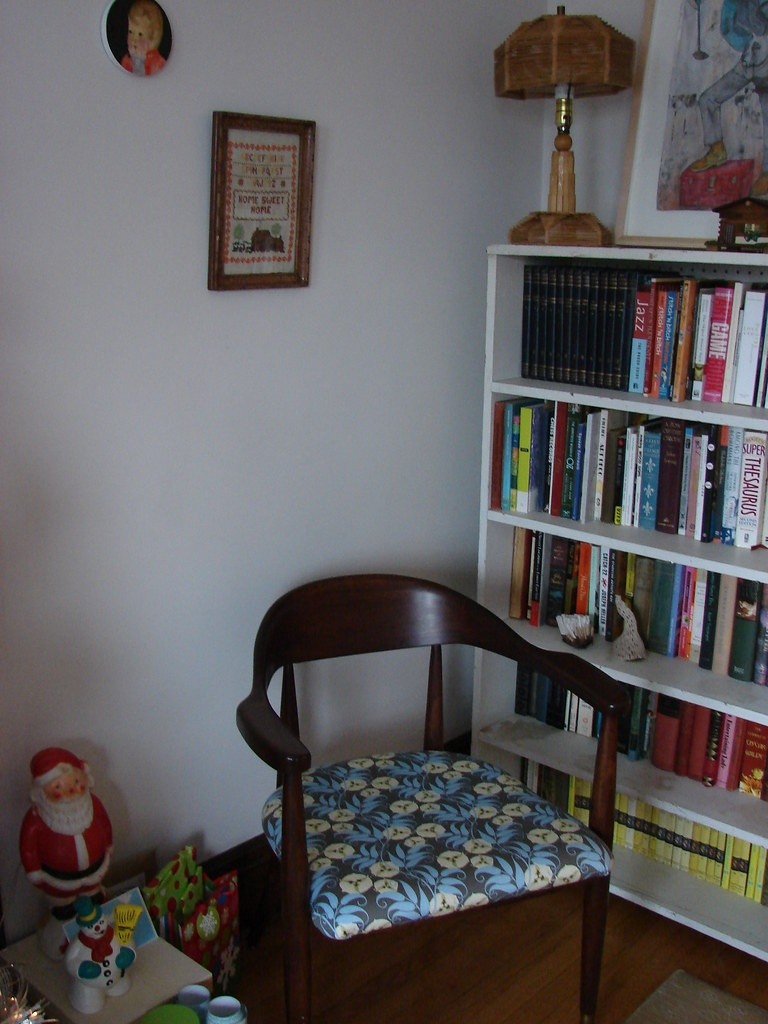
[206,996,247,1024]
[140,1005,200,1024]
[176,984,211,1024]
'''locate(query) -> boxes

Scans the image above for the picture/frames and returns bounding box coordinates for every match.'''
[614,0,768,250]
[205,110,317,289]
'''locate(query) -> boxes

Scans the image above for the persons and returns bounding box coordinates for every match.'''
[18,747,114,921]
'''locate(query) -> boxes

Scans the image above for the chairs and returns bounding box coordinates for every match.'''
[237,572,633,1024]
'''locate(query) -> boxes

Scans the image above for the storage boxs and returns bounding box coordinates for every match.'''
[0,926,215,1024]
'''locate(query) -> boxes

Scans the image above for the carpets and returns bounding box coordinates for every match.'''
[624,966,768,1024]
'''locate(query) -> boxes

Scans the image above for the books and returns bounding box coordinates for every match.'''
[521,265,768,409]
[509,526,768,688]
[491,397,768,550]
[514,662,768,802]
[519,756,768,907]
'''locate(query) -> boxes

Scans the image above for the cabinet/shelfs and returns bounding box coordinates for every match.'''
[471,244,768,966]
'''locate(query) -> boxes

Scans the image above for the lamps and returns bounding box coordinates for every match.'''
[494,5,637,247]
